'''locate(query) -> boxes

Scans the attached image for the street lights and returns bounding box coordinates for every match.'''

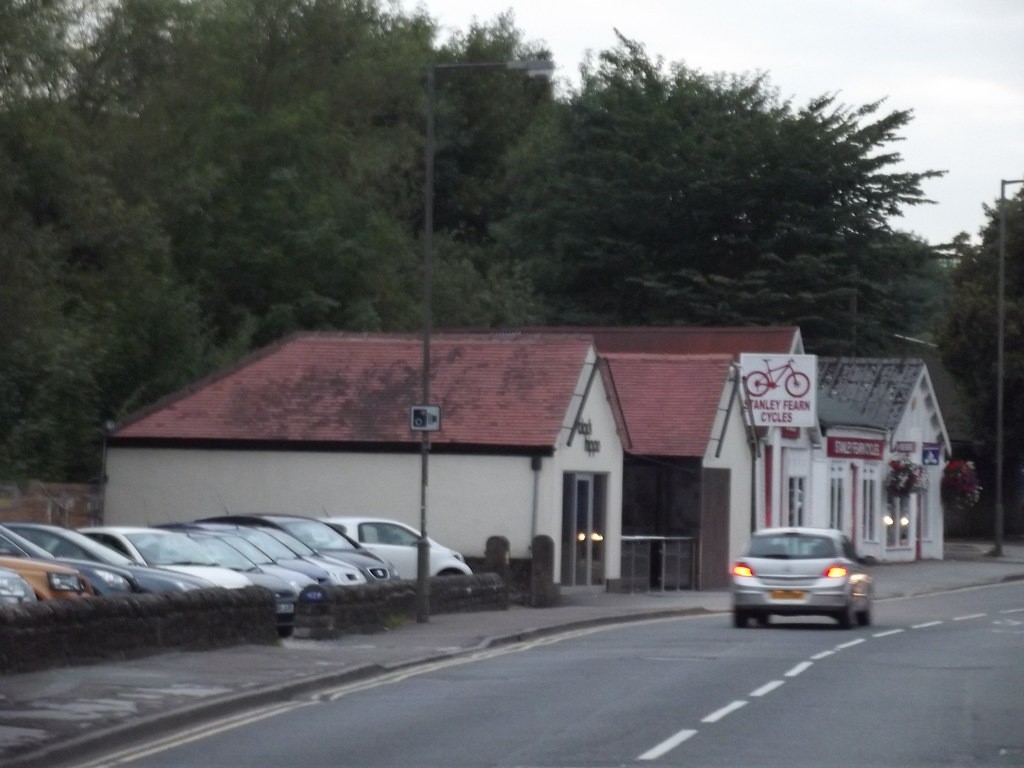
[413,56,556,626]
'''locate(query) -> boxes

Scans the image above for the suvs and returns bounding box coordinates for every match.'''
[0,524,142,600]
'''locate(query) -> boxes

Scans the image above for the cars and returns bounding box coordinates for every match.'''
[46,526,254,599]
[0,554,94,608]
[324,516,474,595]
[4,522,215,606]
[197,513,402,591]
[730,528,872,630]
[159,522,367,637]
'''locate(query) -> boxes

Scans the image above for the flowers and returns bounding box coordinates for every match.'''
[887,459,934,493]
[942,460,983,506]
